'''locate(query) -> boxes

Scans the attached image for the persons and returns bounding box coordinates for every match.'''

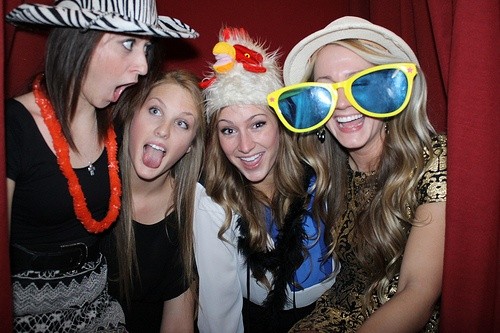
[0,0,447,333]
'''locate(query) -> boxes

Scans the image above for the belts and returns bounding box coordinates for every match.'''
[10,239,99,271]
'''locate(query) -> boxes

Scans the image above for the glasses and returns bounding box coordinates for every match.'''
[266,63,418,133]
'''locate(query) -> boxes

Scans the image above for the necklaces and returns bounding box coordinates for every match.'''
[75,140,96,176]
[32,71,122,233]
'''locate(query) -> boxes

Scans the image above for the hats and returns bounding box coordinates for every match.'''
[7,0,200,39]
[282,16,419,87]
